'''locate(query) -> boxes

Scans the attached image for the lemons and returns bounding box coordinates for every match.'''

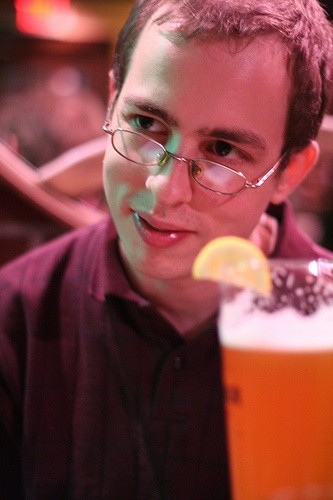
[192,236,272,296]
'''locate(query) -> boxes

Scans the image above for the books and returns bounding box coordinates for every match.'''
[0,134,108,199]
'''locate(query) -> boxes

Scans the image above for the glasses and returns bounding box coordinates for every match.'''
[101,91,289,196]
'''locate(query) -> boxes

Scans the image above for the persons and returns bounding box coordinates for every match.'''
[0,0,333,500]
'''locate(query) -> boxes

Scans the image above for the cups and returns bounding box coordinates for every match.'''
[215,251,333,499]
